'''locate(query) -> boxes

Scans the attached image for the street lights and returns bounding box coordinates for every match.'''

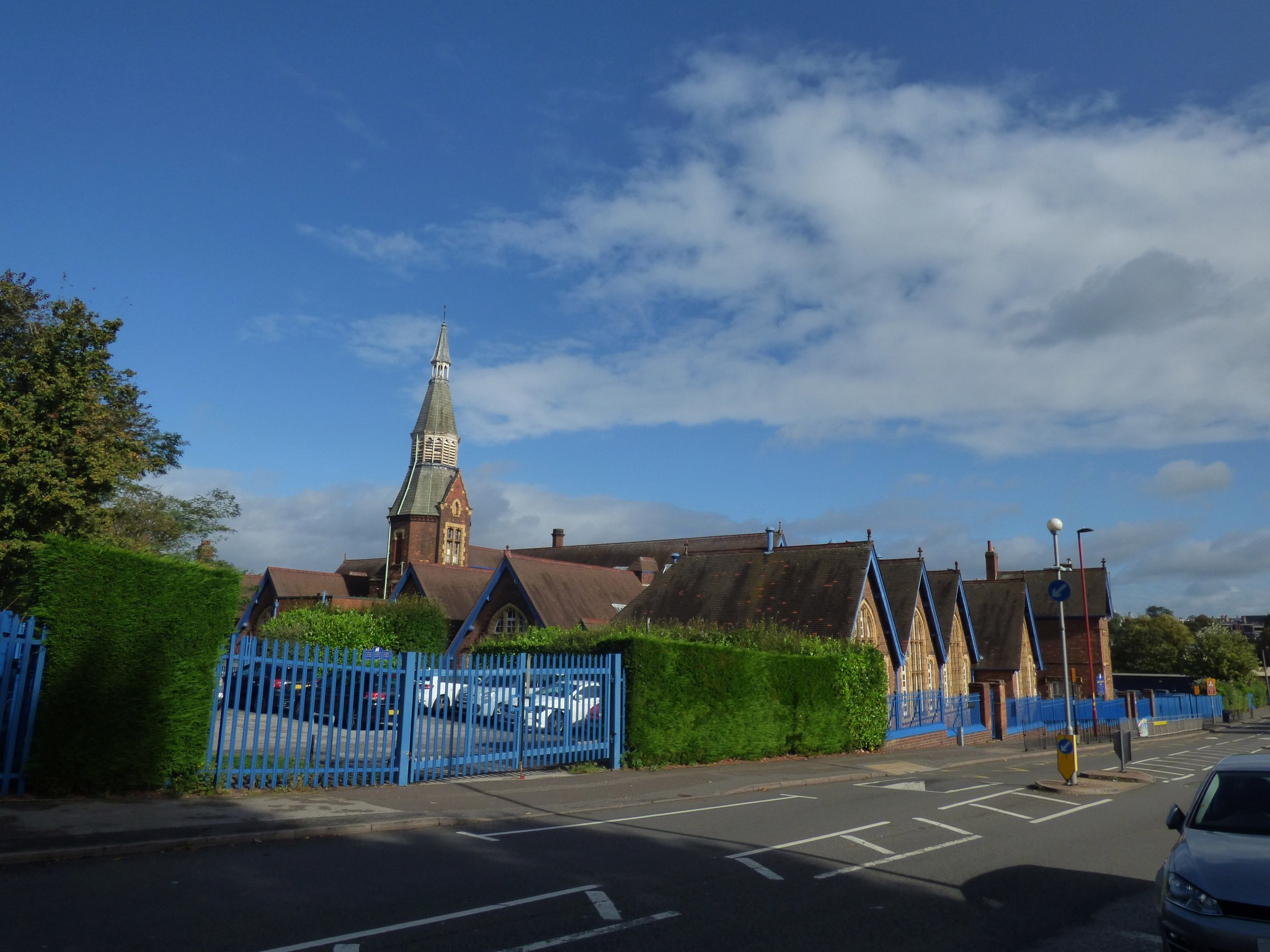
[1076,527,1102,736]
[1047,517,1079,785]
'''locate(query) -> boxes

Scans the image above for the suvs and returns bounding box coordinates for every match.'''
[221,660,319,714]
[293,670,420,731]
[419,676,481,716]
[217,677,225,712]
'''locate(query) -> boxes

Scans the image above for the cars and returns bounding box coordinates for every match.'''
[503,680,600,734]
[1154,753,1270,952]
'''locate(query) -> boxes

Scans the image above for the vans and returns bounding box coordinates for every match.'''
[455,676,565,719]
